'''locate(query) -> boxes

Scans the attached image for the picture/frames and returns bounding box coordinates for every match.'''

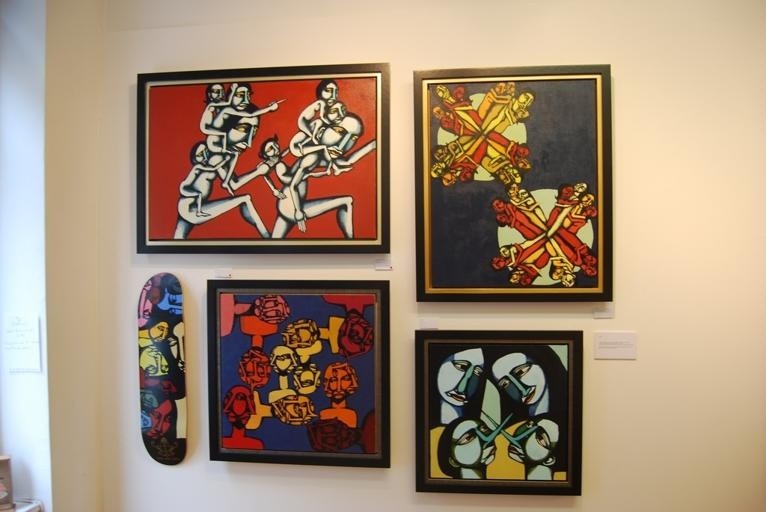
[135,63,391,254]
[412,62,615,306]
[414,327,585,497]
[207,279,392,469]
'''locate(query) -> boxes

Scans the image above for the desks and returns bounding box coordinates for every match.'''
[0,496,45,512]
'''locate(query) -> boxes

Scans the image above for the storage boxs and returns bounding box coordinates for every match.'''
[0,455,12,503]
